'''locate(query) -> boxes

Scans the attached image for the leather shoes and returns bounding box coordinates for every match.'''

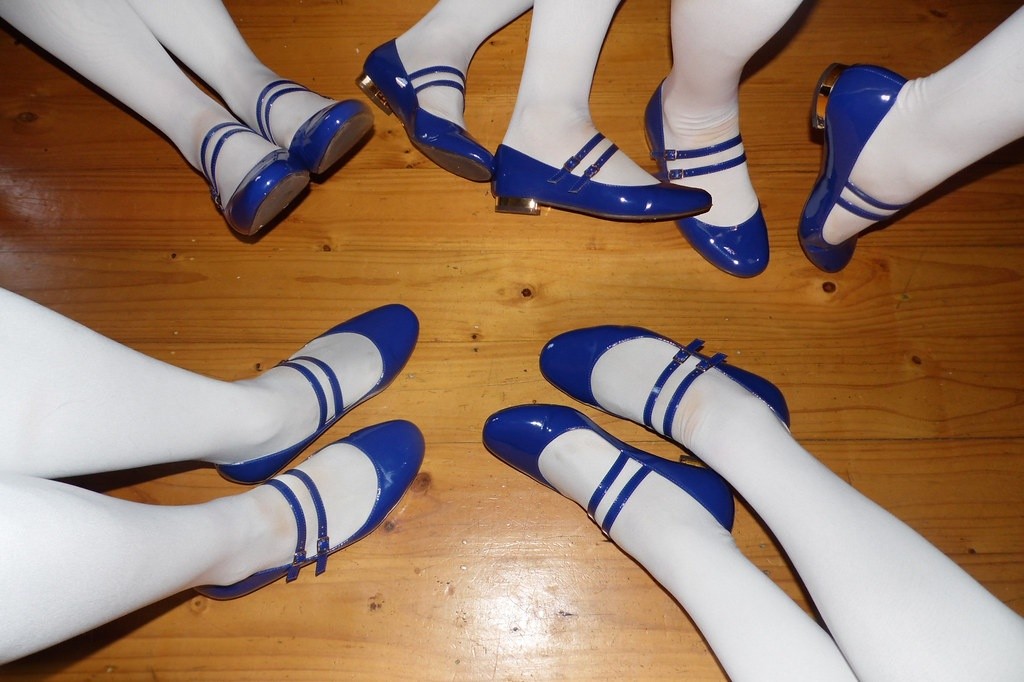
[192,418,425,601]
[200,122,310,237]
[214,303,420,484]
[796,62,911,274]
[482,403,735,537]
[642,76,770,278]
[490,131,712,222]
[354,37,497,183]
[256,79,374,175]
[539,324,790,441]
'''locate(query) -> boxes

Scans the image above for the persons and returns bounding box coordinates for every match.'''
[645,1,1024,280]
[0,0,373,237]
[353,1,711,224]
[0,285,428,663]
[480,322,1024,682]
